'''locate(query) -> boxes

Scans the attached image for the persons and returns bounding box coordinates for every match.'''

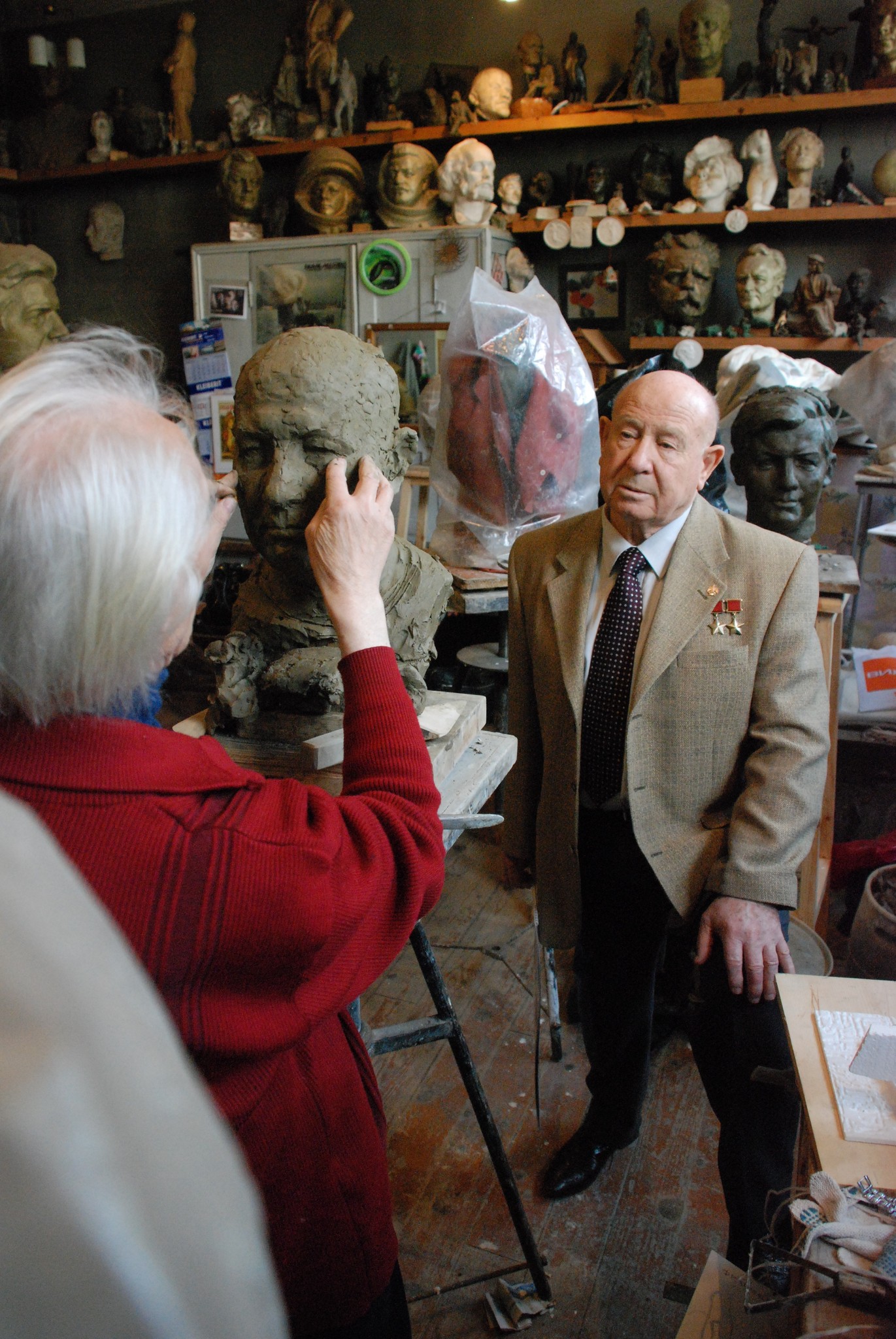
[447,314,582,574]
[498,370,831,1296]
[84,201,124,261]
[0,326,448,1339]
[0,242,71,374]
[202,325,452,742]
[643,230,874,340]
[219,129,853,241]
[729,385,838,543]
[7,0,896,165]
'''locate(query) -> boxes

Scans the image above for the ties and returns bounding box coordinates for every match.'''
[579,547,649,812]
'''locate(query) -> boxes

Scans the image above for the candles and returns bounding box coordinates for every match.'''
[27,36,48,66]
[45,41,57,66]
[67,37,87,69]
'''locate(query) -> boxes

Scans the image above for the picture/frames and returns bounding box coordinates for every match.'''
[561,264,628,330]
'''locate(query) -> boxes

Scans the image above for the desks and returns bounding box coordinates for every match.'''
[795,444,895,934]
[346,728,554,1302]
[774,973,896,1194]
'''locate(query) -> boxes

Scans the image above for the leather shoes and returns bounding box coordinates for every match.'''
[541,1118,642,1199]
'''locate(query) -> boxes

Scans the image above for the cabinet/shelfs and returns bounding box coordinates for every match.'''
[12,87,896,350]
[192,226,516,542]
